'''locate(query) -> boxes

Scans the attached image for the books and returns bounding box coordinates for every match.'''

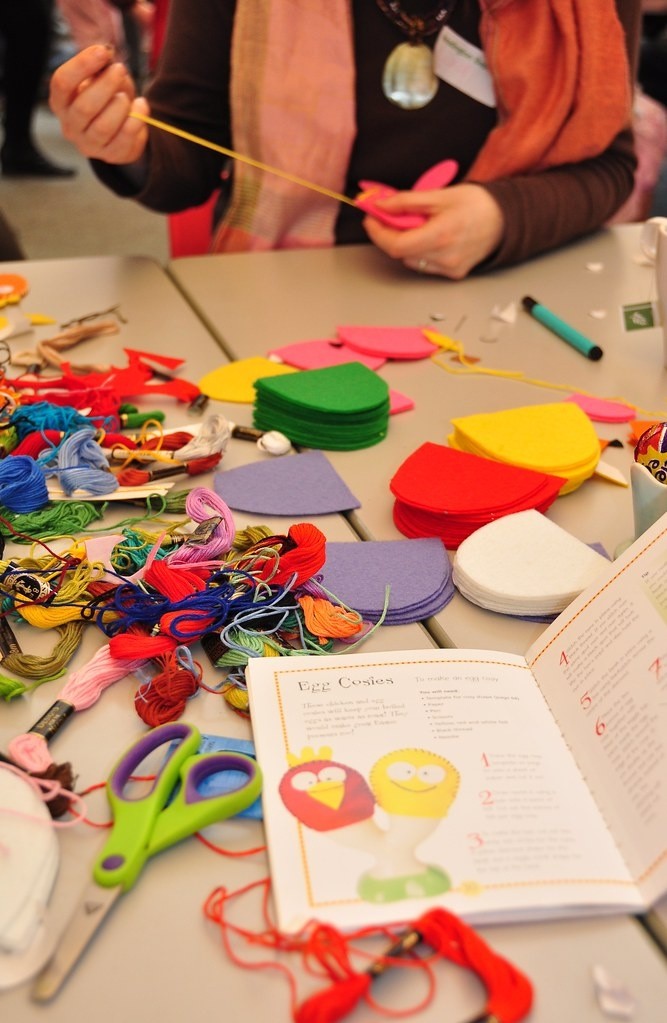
[245,510,667,942]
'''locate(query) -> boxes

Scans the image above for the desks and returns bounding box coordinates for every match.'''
[0,215,667,1022]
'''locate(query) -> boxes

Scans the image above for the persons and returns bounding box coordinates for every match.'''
[1,0,640,280]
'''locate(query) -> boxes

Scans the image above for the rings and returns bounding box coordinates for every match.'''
[417,260,428,274]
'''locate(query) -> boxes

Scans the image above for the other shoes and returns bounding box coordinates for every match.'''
[0,142,72,177]
